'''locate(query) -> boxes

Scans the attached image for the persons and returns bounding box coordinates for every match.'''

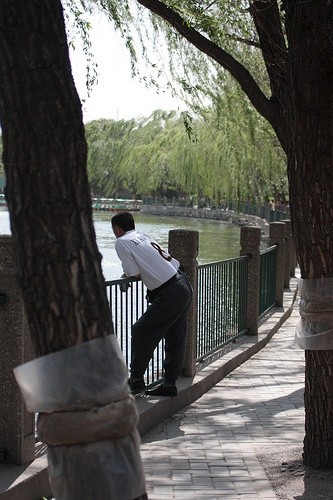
[111,212,194,397]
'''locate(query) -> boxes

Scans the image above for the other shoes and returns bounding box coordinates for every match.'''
[127,377,145,391]
[146,385,177,396]
